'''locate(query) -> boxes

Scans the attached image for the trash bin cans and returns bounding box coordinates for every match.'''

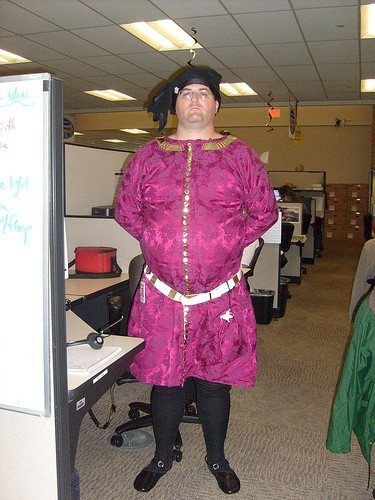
[272,277,291,319]
[249,289,275,324]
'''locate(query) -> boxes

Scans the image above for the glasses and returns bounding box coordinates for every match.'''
[178,89,212,98]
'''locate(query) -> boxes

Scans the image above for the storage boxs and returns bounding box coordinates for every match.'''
[324,183,369,239]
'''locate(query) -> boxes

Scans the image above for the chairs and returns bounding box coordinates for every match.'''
[279,223,295,269]
[111,254,201,447]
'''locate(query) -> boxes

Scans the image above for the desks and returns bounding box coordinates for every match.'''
[65,270,144,500]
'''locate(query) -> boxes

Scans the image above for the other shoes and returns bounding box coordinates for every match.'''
[133,460,173,492]
[205,455,240,494]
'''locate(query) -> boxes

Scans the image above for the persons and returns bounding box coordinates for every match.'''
[276,184,309,224]
[113,66,279,494]
